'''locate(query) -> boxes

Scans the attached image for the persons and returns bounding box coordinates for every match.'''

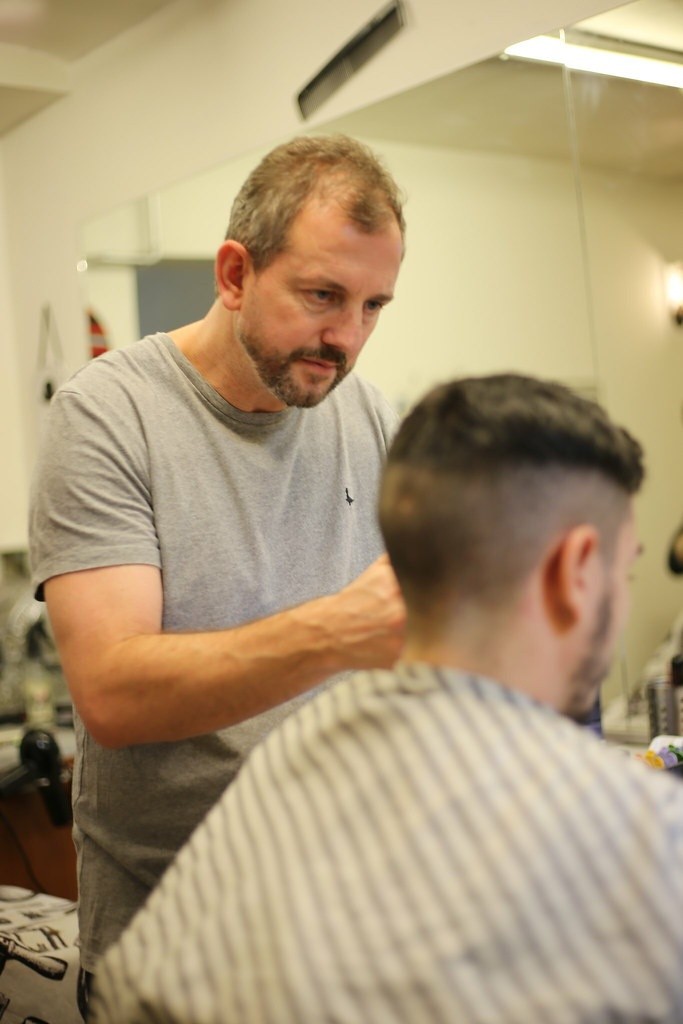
[26,134,410,1024]
[87,376,683,1023]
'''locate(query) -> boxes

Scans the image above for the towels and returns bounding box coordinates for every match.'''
[85,660,683,1022]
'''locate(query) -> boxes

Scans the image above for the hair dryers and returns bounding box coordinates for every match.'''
[0,727,77,830]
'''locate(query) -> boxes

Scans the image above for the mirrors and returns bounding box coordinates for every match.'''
[82,0,681,778]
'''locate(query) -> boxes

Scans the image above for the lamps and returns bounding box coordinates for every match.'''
[665,260,682,322]
